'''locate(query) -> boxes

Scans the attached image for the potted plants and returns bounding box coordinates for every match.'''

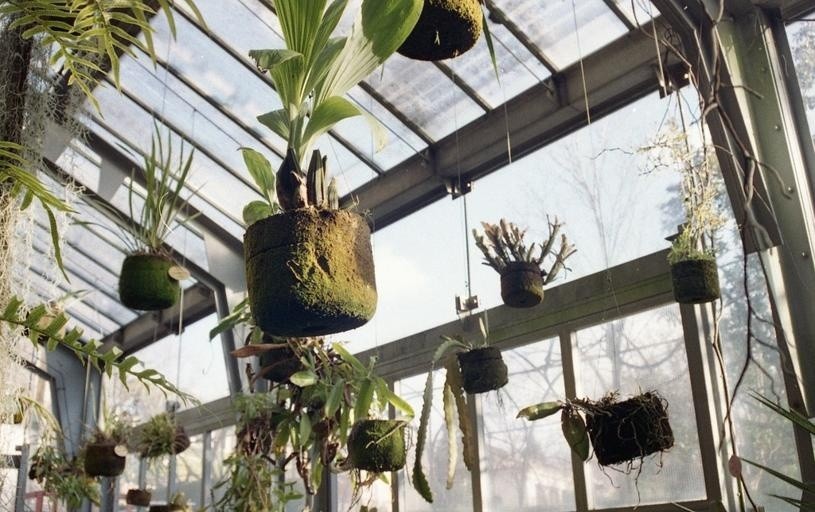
[515,389,674,465]
[109,116,212,311]
[84,394,133,478]
[636,116,733,303]
[412,318,511,507]
[284,342,411,470]
[242,0,423,336]
[470,214,576,306]
